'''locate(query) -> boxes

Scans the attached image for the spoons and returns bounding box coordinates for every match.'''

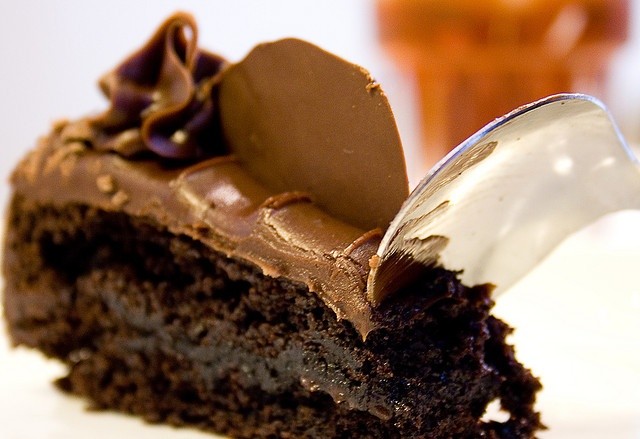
[367,93,639,327]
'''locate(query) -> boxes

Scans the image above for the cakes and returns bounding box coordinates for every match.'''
[1,12,551,438]
